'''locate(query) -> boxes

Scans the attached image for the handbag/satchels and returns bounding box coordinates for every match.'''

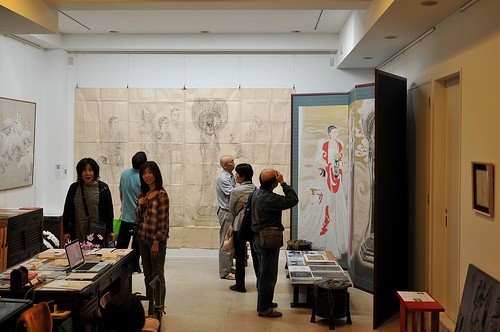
[86,220,107,246]
[223,225,234,252]
[235,187,256,244]
[113,213,125,240]
[259,227,283,249]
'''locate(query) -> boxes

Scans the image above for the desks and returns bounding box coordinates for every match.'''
[396,291,445,332]
[0,247,136,332]
[284,250,353,309]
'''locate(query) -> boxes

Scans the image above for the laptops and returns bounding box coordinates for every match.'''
[64,239,110,273]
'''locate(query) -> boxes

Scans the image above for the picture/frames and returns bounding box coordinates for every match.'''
[470,160,494,218]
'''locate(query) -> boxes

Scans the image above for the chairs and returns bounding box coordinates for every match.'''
[16,300,54,332]
[99,292,160,332]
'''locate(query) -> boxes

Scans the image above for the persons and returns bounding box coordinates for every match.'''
[132,160,170,332]
[246,169,300,318]
[215,154,238,280]
[115,151,148,275]
[228,162,260,292]
[62,157,115,248]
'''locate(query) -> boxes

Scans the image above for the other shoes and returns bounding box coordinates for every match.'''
[272,302,278,308]
[258,310,282,317]
[231,268,235,273]
[221,273,235,280]
[229,284,246,293]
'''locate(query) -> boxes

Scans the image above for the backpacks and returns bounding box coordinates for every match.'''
[103,290,145,331]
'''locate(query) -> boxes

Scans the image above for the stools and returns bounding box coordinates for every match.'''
[285,239,314,269]
[309,279,353,330]
